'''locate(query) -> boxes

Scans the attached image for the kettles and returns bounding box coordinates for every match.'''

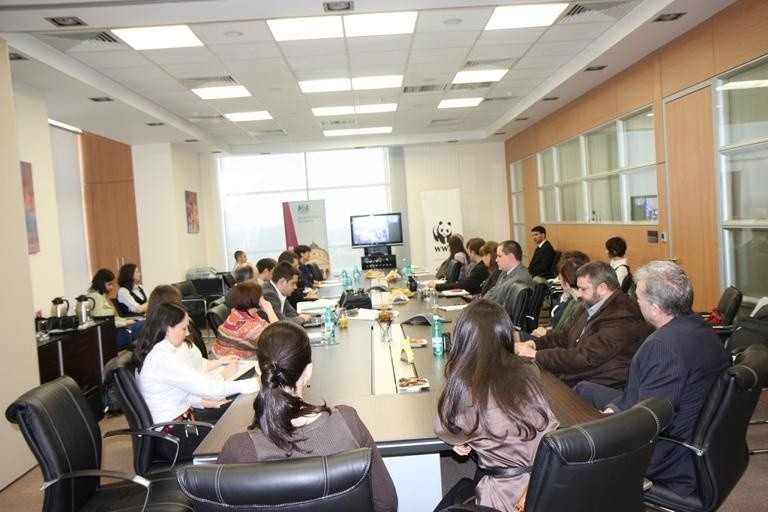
[73,294,96,325]
[50,297,69,319]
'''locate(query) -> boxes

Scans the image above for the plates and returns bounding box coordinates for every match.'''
[365,269,418,322]
[397,376,431,392]
[406,337,428,348]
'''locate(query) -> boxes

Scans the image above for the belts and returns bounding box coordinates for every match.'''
[160,407,195,432]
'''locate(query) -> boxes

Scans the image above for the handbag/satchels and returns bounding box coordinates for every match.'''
[615,264,632,293]
[726,317,768,360]
[339,288,372,309]
[704,308,723,326]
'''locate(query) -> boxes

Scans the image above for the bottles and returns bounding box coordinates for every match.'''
[340,263,361,289]
[323,305,335,340]
[402,258,412,274]
[430,314,445,357]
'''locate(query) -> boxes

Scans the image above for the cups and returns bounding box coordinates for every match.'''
[337,308,351,328]
[420,284,438,305]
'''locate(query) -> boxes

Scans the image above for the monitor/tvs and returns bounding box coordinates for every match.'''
[350,212,403,249]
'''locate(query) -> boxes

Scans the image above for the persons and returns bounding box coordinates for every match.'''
[116,264,149,317]
[428,225,645,391]
[430,298,562,512]
[219,322,399,512]
[89,268,146,348]
[571,260,732,498]
[137,246,318,461]
